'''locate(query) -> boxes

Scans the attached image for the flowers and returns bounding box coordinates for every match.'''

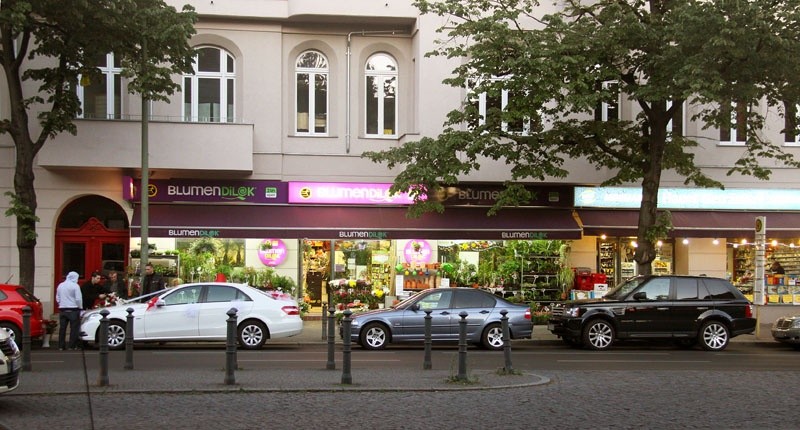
[329,277,390,310]
[44,319,58,334]
[182,239,316,317]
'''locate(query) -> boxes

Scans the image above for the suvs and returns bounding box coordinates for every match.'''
[547,275,758,352]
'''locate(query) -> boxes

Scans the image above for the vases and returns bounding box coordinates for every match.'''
[42,334,49,347]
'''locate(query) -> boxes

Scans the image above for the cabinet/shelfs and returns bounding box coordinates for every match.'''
[130,255,180,278]
[735,252,800,282]
[495,255,562,303]
[600,246,673,288]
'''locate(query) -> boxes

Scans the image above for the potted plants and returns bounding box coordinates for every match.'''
[131,244,180,276]
[451,239,575,326]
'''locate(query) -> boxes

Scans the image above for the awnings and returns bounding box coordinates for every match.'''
[130,202,582,240]
[575,209,800,238]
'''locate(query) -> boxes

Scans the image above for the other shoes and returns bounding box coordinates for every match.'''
[73,347,77,351]
[58,348,64,350]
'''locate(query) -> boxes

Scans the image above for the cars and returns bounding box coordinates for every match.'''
[341,286,534,352]
[0,326,24,396]
[771,312,800,351]
[76,281,303,352]
[0,283,44,352]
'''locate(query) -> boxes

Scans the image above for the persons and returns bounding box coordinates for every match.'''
[56,264,165,350]
[769,258,784,274]
